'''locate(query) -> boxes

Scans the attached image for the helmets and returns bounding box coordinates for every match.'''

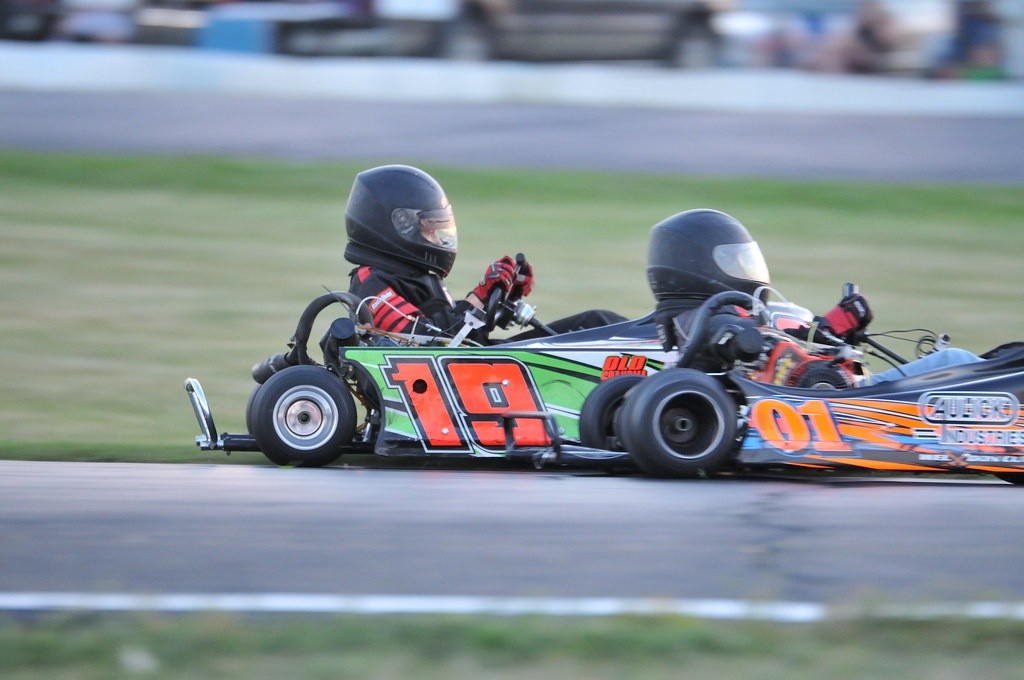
[345,166,457,276]
[646,207,770,303]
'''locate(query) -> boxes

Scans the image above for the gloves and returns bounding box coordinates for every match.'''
[473,256,534,306]
[815,294,873,339]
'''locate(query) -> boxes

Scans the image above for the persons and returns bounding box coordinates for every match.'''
[343,164,630,347]
[646,208,988,390]
